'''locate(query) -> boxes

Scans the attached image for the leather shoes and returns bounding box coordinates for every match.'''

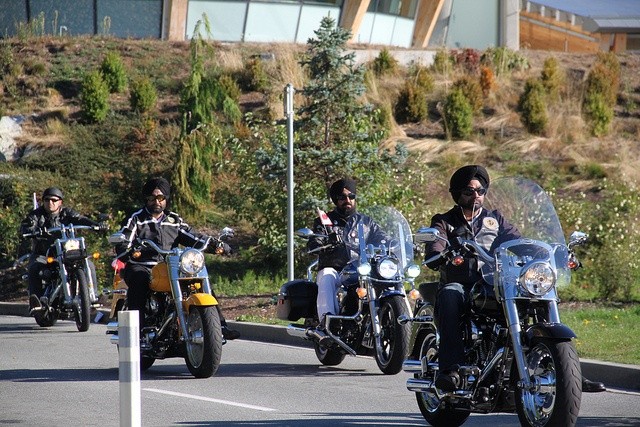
[221,326,240,340]
[29,294,48,325]
[435,372,462,390]
[581,375,604,393]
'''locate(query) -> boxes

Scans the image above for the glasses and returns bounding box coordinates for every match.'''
[43,197,60,203]
[461,187,485,196]
[336,192,356,199]
[145,194,166,202]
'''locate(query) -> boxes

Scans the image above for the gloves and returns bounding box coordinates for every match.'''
[312,242,332,255]
[24,226,37,236]
[208,238,231,256]
[448,249,466,262]
[571,254,580,271]
[408,243,422,258]
[94,222,108,233]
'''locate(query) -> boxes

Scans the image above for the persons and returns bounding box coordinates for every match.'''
[307,180,423,332]
[424,165,606,394]
[115,179,242,341]
[20,188,109,314]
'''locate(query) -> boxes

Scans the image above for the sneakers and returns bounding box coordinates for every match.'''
[320,312,341,335]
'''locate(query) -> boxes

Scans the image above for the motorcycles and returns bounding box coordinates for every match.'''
[275,206,421,375]
[105,226,240,379]
[396,175,589,427]
[13,222,110,332]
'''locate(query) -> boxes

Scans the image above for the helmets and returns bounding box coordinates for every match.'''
[42,188,63,200]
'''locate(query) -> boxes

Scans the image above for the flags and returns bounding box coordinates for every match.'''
[316,207,333,226]
